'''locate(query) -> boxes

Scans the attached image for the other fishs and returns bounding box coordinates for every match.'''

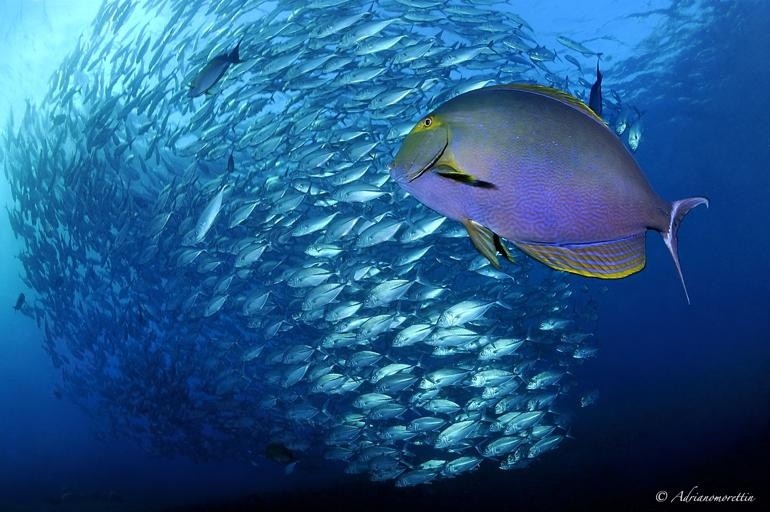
[387,81,711,308]
[0,0,647,488]
